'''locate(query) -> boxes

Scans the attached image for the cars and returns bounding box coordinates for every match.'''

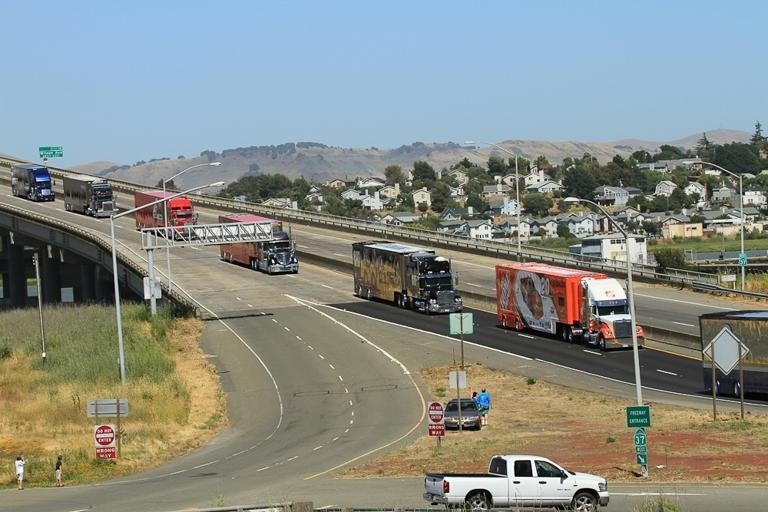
[445,398,481,430]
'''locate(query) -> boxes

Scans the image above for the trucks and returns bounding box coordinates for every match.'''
[63,174,120,218]
[9,163,56,202]
[218,211,299,275]
[352,241,463,314]
[494,261,645,351]
[135,190,199,241]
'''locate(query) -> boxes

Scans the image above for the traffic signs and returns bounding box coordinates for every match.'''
[626,407,650,427]
[39,146,63,158]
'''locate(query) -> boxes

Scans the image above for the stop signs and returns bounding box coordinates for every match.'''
[95,424,116,459]
[428,402,445,437]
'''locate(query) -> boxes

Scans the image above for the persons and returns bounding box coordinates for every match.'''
[55,455,63,486]
[477,387,493,425]
[471,390,484,424]
[15,456,27,490]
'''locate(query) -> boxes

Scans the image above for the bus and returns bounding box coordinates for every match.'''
[581,235,647,267]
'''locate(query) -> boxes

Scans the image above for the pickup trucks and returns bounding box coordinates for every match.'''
[424,454,609,511]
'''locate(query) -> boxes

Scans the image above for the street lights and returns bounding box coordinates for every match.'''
[464,138,521,254]
[163,161,223,292]
[109,181,225,383]
[563,196,644,406]
[683,160,745,292]
